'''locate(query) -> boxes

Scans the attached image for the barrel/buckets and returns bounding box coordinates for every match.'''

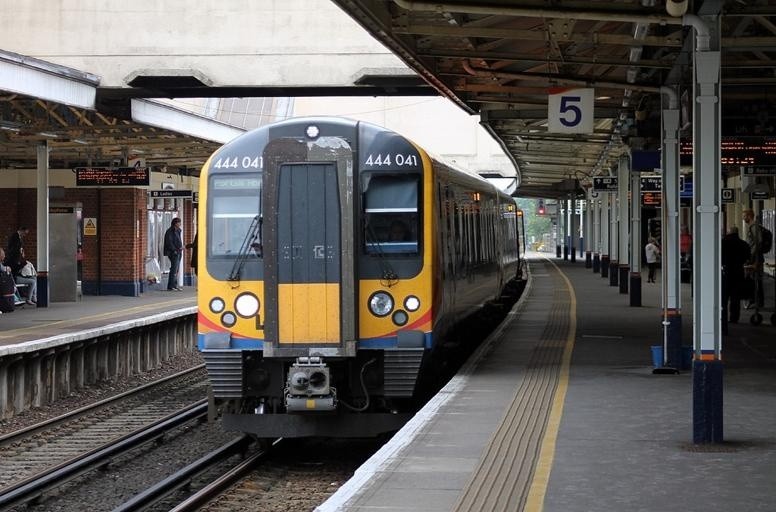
[650,344,679,371]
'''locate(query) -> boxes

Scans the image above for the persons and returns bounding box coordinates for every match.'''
[722,226,752,323]
[645,237,660,283]
[163,217,185,292]
[0,224,37,314]
[180,232,198,292]
[742,208,766,308]
[76,244,82,281]
[680,224,692,261]
[387,218,410,242]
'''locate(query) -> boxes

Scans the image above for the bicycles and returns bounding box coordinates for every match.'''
[743,264,759,309]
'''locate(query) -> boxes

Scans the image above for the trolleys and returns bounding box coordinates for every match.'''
[749,264,776,327]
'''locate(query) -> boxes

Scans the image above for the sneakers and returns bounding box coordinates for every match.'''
[168,286,183,291]
[27,298,37,304]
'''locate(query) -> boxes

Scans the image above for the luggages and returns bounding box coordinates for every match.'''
[0,256,14,313]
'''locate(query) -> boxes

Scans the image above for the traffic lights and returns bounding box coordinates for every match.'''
[537,201,545,215]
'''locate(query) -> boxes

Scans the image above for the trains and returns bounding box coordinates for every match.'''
[197,115,524,454]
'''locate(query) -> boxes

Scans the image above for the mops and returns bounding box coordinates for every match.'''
[652,189,680,375]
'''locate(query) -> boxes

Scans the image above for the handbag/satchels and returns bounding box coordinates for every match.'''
[750,223,772,253]
[21,260,36,276]
